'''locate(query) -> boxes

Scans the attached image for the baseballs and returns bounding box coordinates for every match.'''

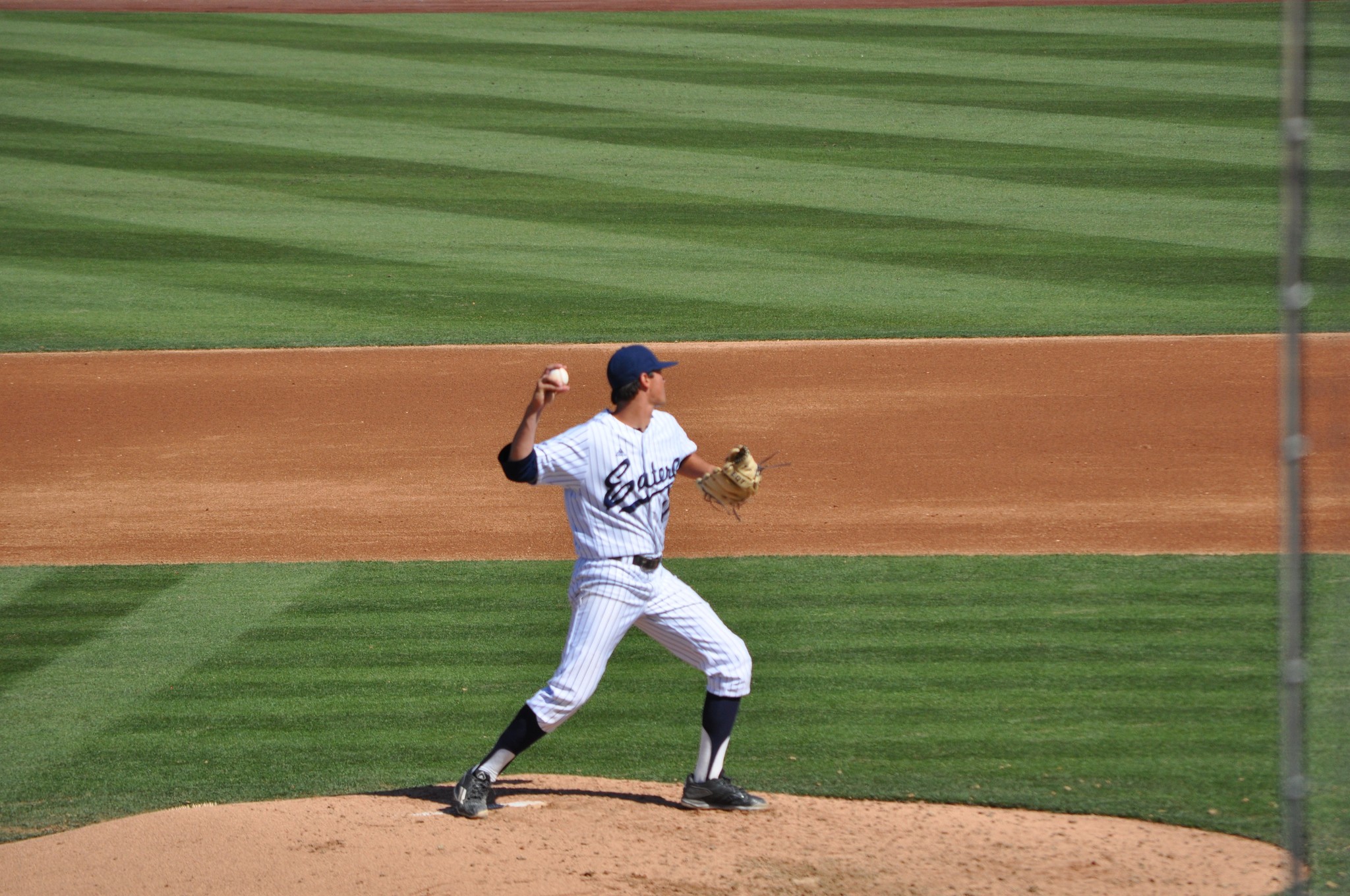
[549,367,569,385]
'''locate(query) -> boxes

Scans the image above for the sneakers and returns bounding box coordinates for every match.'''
[680,769,768,811]
[451,766,495,819]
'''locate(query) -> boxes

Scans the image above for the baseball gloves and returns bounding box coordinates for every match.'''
[695,443,790,523]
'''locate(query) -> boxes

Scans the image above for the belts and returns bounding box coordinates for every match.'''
[611,552,661,569]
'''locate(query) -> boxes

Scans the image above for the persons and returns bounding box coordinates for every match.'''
[452,346,771,823]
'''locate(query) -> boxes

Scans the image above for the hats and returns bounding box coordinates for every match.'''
[606,346,679,389]
